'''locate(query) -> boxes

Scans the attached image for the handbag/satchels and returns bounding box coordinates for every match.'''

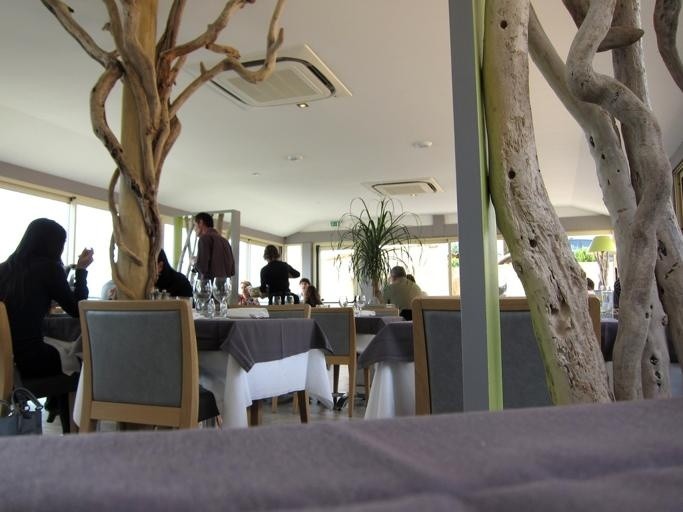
[0,387,44,436]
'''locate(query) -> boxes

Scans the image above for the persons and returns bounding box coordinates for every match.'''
[586,274,621,307]
[157,213,428,315]
[0,218,94,434]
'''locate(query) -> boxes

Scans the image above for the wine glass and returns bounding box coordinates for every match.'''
[339,294,366,315]
[191,277,232,319]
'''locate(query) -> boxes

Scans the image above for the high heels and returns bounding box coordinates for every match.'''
[43,397,61,423]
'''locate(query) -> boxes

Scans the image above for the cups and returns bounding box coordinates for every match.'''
[273,296,293,305]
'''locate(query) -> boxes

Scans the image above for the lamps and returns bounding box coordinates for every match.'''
[588,235,618,261]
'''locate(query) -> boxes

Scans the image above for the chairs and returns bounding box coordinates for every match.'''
[261,302,361,423]
[72,296,223,433]
[409,296,604,413]
[0,300,71,431]
